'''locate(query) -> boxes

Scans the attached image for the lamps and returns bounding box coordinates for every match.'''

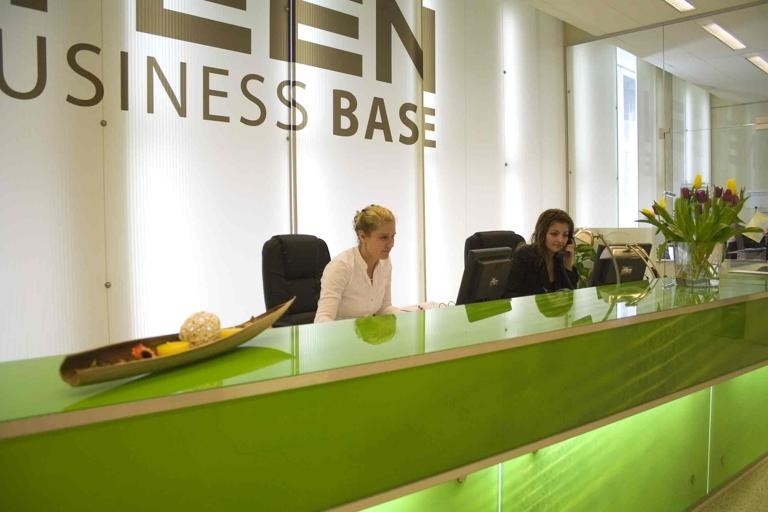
[574,229,620,282]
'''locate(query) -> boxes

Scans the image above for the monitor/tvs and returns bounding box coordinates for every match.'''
[456,246,513,304]
[590,244,652,286]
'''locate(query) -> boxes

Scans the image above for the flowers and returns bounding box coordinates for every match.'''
[634,175,765,281]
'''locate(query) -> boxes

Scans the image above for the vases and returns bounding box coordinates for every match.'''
[672,240,723,288]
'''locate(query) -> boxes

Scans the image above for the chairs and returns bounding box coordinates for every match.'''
[263,234,333,328]
[464,230,526,267]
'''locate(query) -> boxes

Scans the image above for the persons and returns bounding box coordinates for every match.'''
[312,203,416,323]
[498,208,580,299]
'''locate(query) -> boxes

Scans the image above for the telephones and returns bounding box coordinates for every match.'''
[566,235,575,253]
